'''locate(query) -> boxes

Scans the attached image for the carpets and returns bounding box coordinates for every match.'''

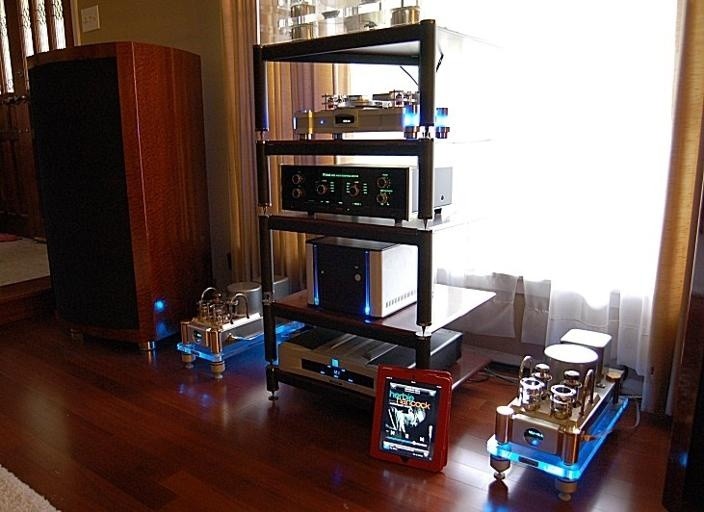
[1,465,56,512]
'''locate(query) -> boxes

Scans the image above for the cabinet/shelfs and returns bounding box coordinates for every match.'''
[251,20,499,404]
[27,42,215,352]
